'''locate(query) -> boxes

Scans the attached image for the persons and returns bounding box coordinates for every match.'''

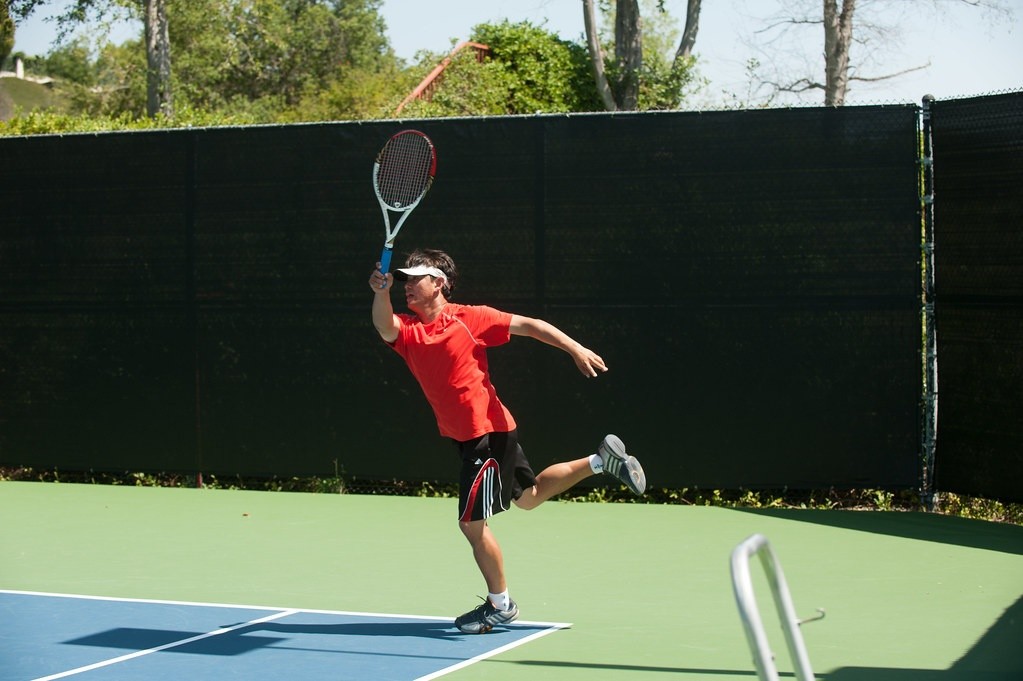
[369,249,647,635]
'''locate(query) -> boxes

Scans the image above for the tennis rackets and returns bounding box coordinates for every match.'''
[372,128,436,289]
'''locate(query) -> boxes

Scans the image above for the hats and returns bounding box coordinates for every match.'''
[391,261,454,291]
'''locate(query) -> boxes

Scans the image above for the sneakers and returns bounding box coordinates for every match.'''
[454,595,520,634]
[597,434,646,495]
